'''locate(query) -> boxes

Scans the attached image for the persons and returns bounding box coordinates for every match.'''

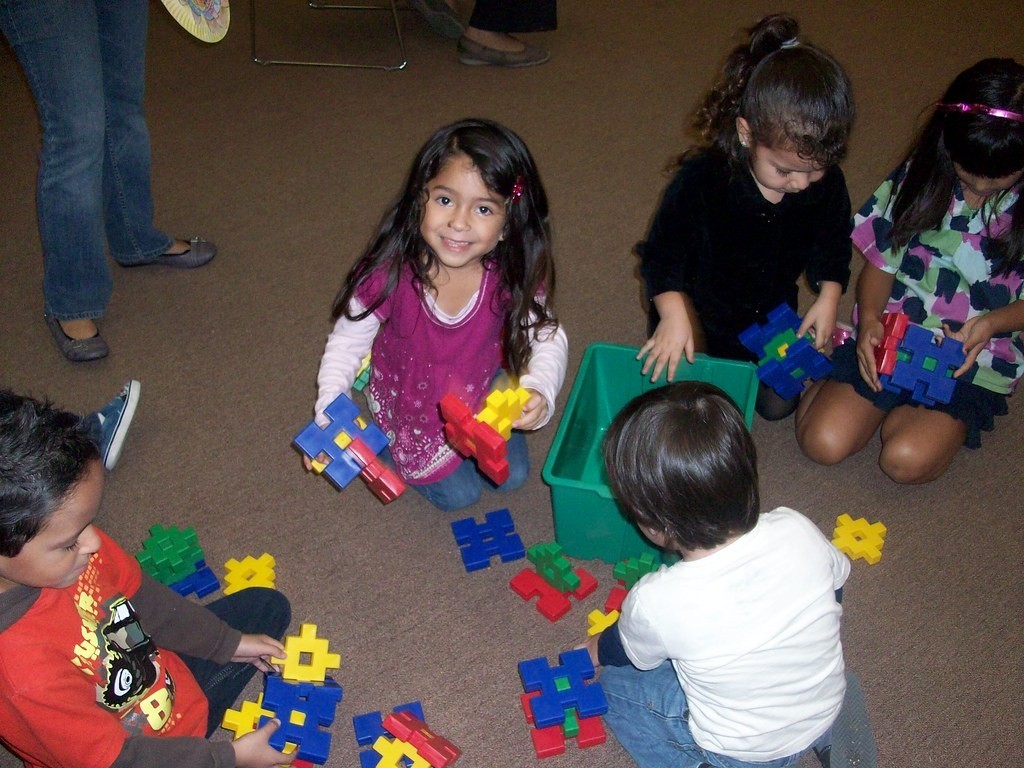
[84,378,143,472]
[0,0,216,361]
[302,116,569,513]
[410,0,559,69]
[633,7,864,424]
[792,58,1024,486]
[573,379,879,768]
[0,390,300,768]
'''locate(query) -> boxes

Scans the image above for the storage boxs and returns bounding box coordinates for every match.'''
[540,340,761,562]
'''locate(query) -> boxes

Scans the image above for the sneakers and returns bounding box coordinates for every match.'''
[43,312,109,362]
[458,33,552,67]
[415,0,466,39]
[97,379,141,470]
[117,237,218,268]
